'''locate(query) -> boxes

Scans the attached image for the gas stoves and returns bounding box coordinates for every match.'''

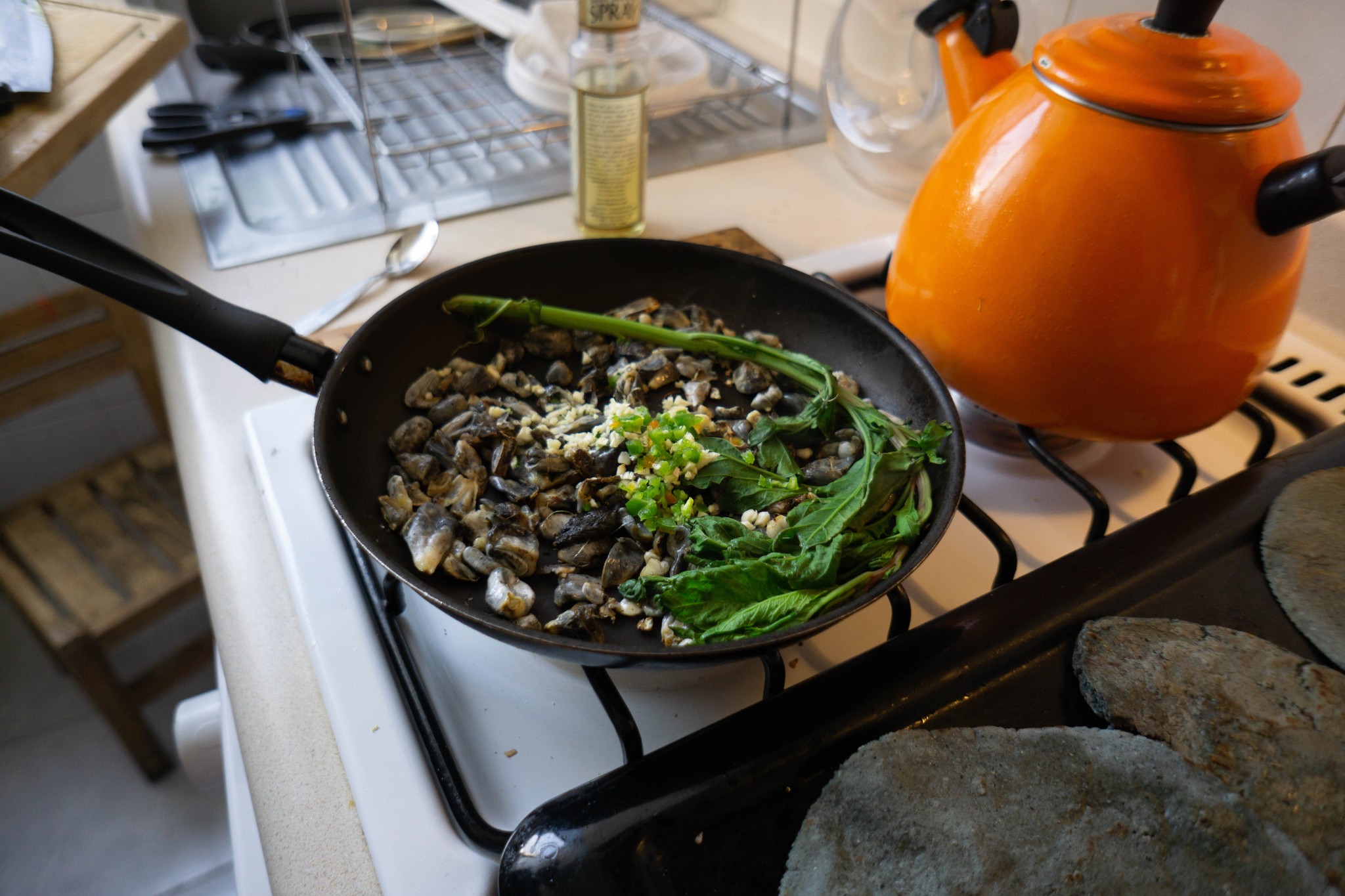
[205,191,1345,896]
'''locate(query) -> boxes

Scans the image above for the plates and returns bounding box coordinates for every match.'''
[505,19,713,121]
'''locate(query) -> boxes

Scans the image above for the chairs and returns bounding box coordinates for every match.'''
[0,286,211,784]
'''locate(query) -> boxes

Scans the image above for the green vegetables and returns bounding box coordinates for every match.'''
[447,293,954,649]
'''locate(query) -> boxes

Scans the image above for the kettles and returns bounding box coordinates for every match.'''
[873,1,1344,448]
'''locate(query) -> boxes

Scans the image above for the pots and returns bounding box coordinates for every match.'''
[0,190,970,667]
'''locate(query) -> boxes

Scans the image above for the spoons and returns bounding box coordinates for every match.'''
[290,214,438,338]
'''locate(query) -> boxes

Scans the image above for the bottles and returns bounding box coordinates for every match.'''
[565,1,654,238]
